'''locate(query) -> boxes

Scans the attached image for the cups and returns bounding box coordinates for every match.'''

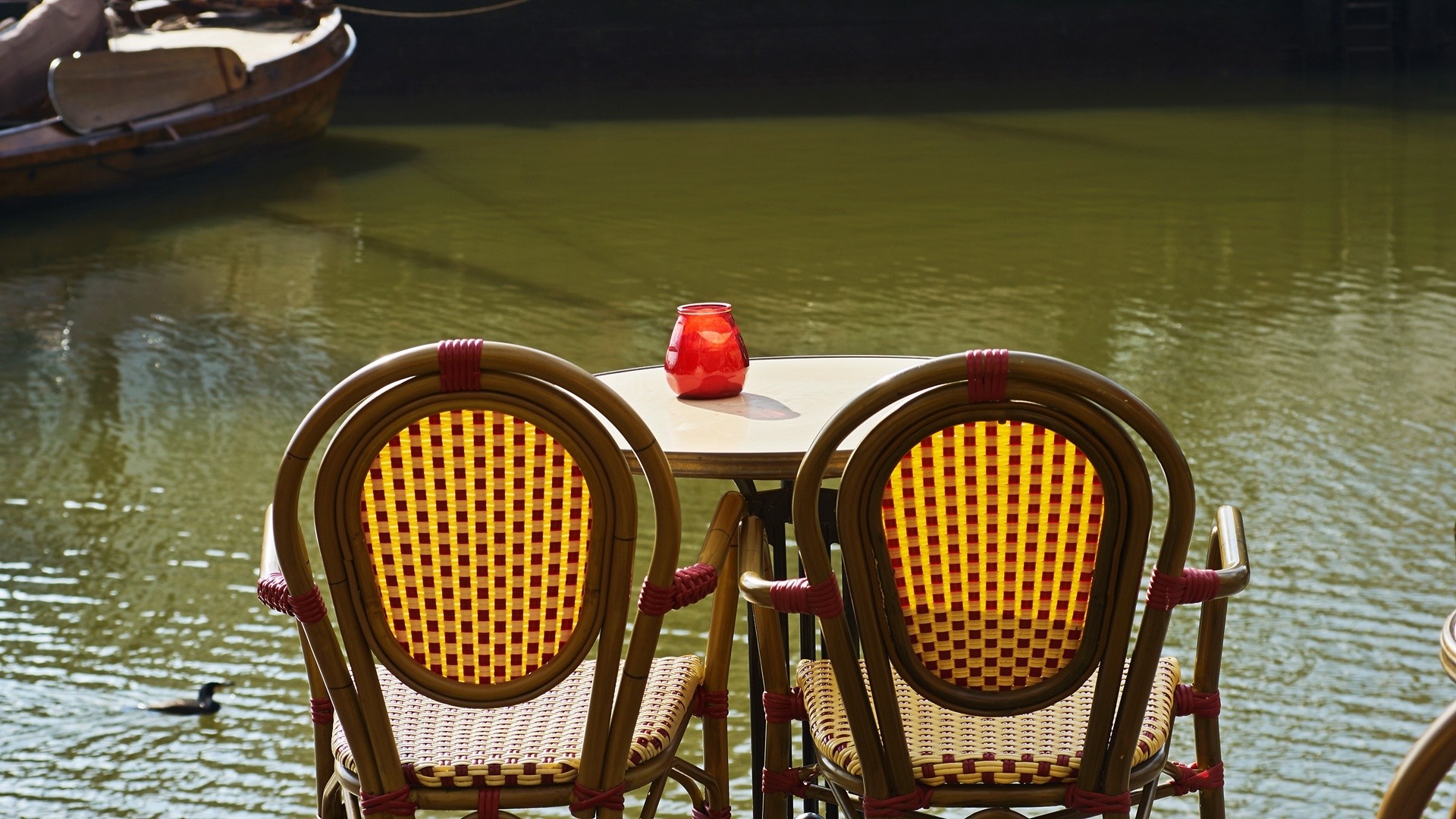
[664,302,749,400]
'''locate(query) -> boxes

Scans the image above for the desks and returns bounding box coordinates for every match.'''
[564,358,969,819]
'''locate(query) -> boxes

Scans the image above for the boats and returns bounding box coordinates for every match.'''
[0,1,358,207]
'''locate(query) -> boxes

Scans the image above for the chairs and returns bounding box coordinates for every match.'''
[260,336,744,818]
[738,346,1250,818]
